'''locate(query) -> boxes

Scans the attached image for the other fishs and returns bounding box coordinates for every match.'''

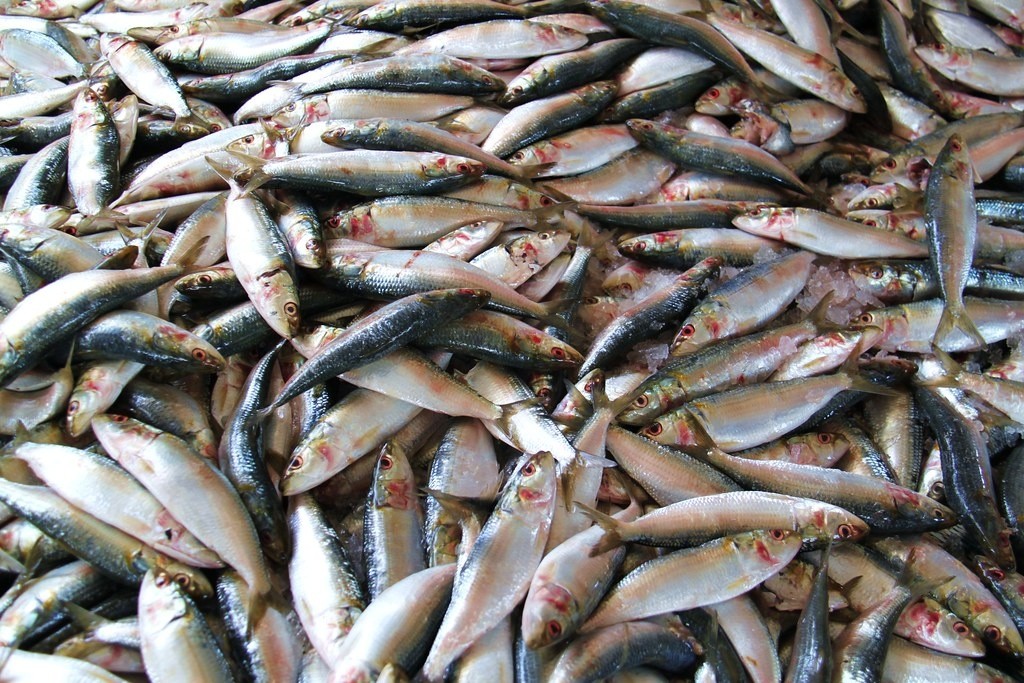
[0,0,1024,683]
